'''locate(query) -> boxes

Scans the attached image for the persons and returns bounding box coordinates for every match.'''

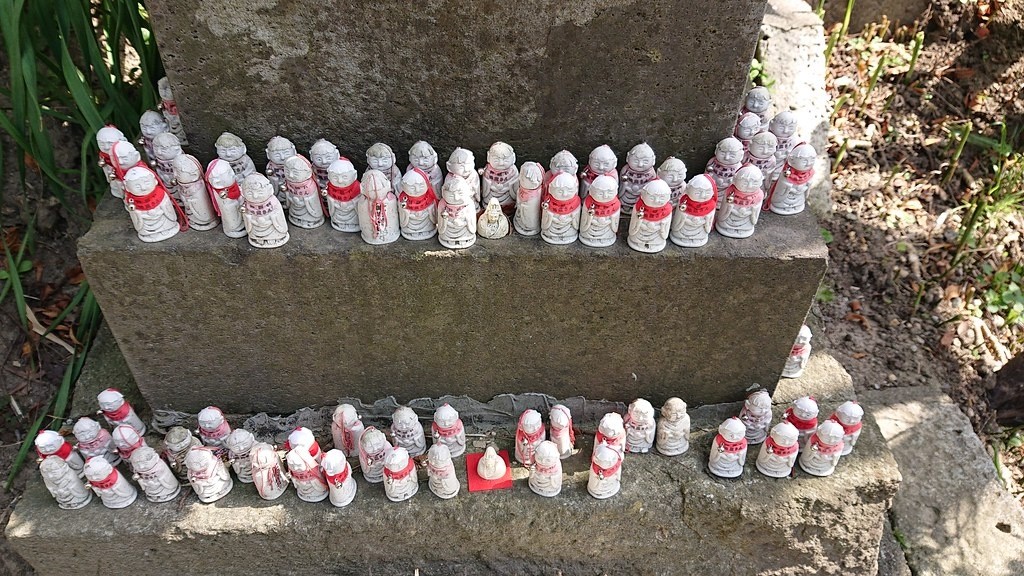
[33,385,864,509]
[95,76,816,254]
[781,324,812,378]
